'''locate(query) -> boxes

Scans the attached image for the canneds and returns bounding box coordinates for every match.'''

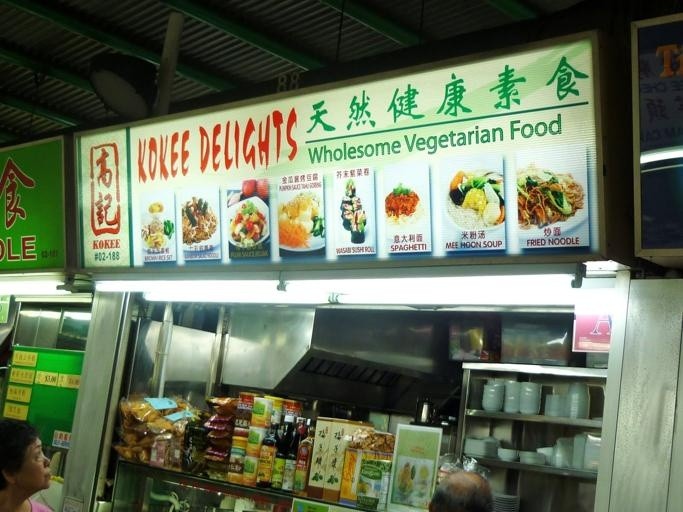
[228,391,304,486]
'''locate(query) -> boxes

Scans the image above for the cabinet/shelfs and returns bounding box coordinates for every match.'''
[454,362,608,512]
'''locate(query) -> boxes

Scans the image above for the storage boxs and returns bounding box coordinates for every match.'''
[447,312,573,365]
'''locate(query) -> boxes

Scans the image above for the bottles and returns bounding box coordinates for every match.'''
[256,415,315,492]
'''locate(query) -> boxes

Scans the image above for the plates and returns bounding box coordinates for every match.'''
[141,196,324,252]
[464,438,501,459]
[517,200,590,238]
[492,494,520,512]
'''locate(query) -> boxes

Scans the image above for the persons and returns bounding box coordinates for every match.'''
[427,471,494,512]
[0,418,55,512]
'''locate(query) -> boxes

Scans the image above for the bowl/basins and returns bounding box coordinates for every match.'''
[482,381,591,420]
[497,447,553,466]
[444,195,505,235]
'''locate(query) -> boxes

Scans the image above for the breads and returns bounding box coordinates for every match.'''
[115,398,186,463]
